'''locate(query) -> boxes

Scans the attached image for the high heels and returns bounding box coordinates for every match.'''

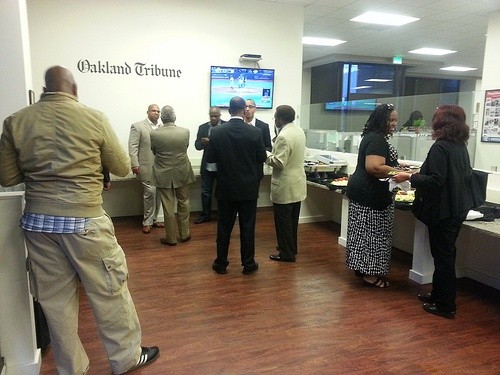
[361,276,390,287]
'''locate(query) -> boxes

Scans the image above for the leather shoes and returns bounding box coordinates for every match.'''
[418,292,434,303]
[423,303,456,319]
[270,255,283,261]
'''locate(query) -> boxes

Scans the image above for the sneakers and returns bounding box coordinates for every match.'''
[130,345,160,369]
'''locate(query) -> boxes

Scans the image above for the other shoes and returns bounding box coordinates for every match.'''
[195,216,211,223]
[160,238,177,246]
[243,262,259,274]
[211,262,228,274]
[153,222,164,228]
[180,235,191,242]
[142,225,152,233]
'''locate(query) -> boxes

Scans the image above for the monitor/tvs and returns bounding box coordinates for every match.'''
[209,66,274,109]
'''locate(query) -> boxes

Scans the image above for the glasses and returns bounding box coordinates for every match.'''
[382,103,394,110]
[245,104,255,108]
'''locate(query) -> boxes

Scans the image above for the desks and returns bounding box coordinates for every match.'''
[307,179,500,285]
[103,165,271,217]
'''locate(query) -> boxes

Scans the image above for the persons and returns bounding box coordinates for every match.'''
[243,99,272,179]
[128,104,165,233]
[345,104,411,287]
[211,96,267,275]
[194,106,228,223]
[149,105,197,247]
[0,65,160,375]
[265,105,307,262]
[394,105,482,319]
[400,111,423,131]
